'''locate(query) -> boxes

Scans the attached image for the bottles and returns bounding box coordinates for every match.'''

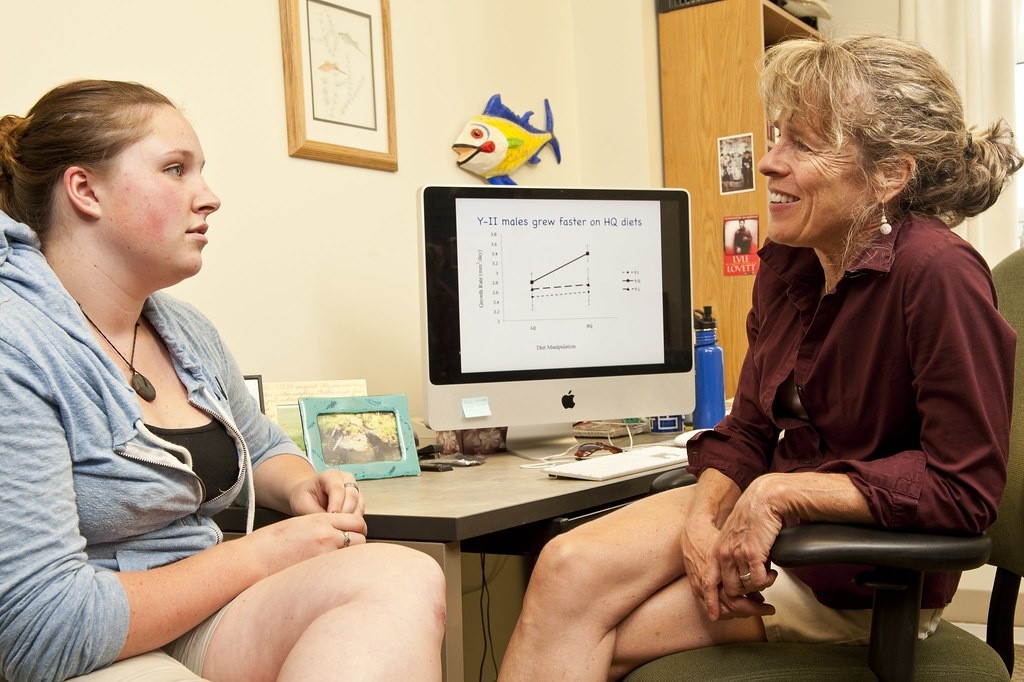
[692,306,725,430]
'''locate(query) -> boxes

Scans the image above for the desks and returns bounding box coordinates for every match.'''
[207,424,692,682]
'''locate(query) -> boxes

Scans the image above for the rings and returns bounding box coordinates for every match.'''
[343,531,350,547]
[344,482,359,491]
[739,573,751,580]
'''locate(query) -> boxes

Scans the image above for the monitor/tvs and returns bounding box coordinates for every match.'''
[420,184,697,466]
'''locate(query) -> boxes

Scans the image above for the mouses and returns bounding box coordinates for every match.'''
[674,428,713,447]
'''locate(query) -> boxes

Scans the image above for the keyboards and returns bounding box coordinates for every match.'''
[542,446,688,481]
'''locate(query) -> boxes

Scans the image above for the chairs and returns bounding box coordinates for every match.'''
[589,244,1024,682]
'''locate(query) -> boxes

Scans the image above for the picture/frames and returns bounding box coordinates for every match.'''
[297,394,424,482]
[280,0,397,173]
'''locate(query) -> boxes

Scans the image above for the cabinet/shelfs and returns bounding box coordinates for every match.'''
[658,0,827,405]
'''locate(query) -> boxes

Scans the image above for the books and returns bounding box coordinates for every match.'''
[545,445,689,482]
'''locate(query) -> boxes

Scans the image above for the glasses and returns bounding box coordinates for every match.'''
[573,441,623,460]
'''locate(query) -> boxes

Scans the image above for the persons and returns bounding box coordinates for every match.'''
[720,150,752,190]
[0,80,449,682]
[497,34,1024,682]
[734,219,752,255]
[331,423,347,464]
[361,426,389,462]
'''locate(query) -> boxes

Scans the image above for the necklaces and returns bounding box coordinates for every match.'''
[77,302,156,402]
